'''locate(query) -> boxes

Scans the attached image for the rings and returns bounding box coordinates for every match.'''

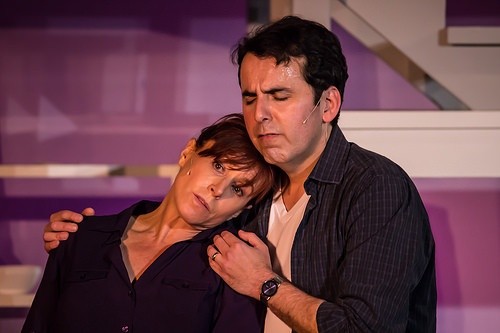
[212,251,216,259]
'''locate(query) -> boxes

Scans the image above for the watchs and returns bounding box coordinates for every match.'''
[259,275,289,307]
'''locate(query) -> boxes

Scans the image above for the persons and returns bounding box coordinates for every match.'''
[43,15,438,333]
[20,113,290,333]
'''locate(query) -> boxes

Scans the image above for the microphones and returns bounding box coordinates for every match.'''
[303,91,325,123]
[187,157,192,175]
